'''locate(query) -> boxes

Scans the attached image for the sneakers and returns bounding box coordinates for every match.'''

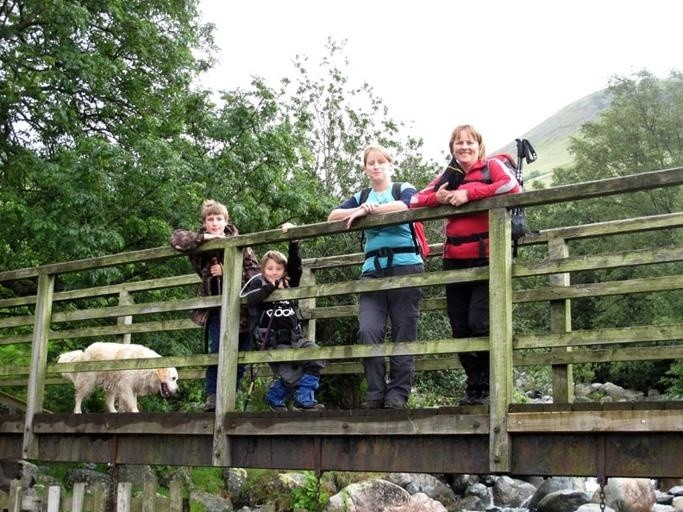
[292,400,325,412]
[384,400,403,409]
[459,391,489,406]
[361,400,384,409]
[203,394,216,412]
[265,397,288,413]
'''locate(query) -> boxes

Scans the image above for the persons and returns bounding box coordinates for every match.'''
[246,222,328,411]
[170,199,259,413]
[409,124,523,407]
[327,144,426,410]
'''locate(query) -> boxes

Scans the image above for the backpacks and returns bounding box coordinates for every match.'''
[445,153,531,245]
[359,182,430,260]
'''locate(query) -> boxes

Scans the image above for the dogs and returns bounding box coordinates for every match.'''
[57,341,181,413]
